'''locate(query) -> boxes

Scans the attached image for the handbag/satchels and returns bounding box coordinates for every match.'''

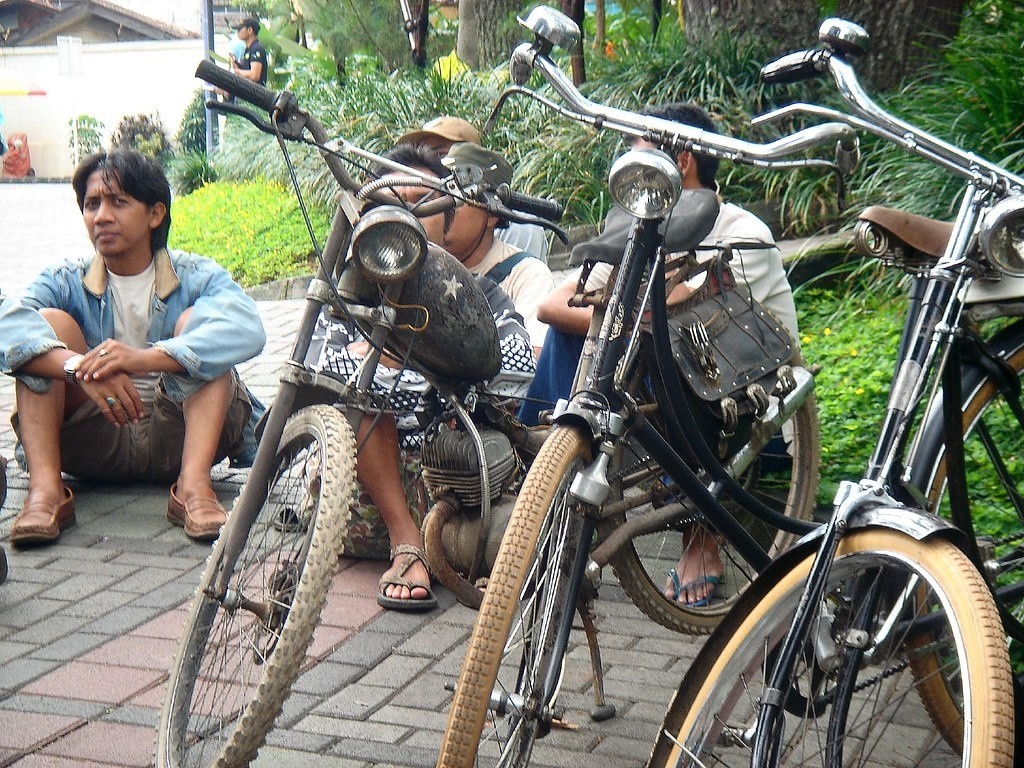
[644,285,800,463]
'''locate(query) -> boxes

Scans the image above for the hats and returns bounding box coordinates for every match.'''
[442,142,514,229]
[395,116,483,147]
[232,19,260,30]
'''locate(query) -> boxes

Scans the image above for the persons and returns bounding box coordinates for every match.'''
[273,116,549,531]
[321,144,556,608]
[518,102,801,607]
[0,149,266,543]
[231,18,267,86]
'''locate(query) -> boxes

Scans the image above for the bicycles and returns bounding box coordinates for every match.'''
[652,13,1024,768]
[154,61,569,768]
[434,2,863,766]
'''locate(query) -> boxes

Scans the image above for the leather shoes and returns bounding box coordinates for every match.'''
[9,487,77,543]
[167,483,229,540]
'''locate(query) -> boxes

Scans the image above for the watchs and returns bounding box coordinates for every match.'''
[63,354,83,385]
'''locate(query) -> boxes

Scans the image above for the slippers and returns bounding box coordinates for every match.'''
[664,567,721,606]
[377,543,439,610]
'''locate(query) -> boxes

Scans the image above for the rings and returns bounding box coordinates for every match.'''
[100,349,108,356]
[106,396,122,405]
